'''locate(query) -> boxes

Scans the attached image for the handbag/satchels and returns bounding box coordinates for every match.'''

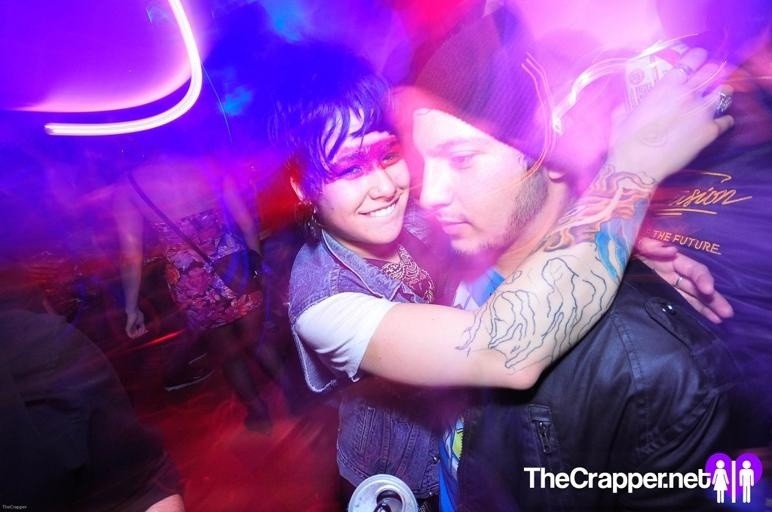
[211,248,263,294]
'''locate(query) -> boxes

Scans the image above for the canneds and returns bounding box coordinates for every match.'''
[346,473,420,512]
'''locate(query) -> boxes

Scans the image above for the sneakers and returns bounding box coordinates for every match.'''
[245,415,273,432]
[290,393,317,414]
[162,349,214,393]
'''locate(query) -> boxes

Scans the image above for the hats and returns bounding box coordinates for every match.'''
[407,7,577,182]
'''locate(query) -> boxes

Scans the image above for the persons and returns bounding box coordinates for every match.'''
[266,0,772,512]
[0,309,185,512]
[112,104,306,431]
